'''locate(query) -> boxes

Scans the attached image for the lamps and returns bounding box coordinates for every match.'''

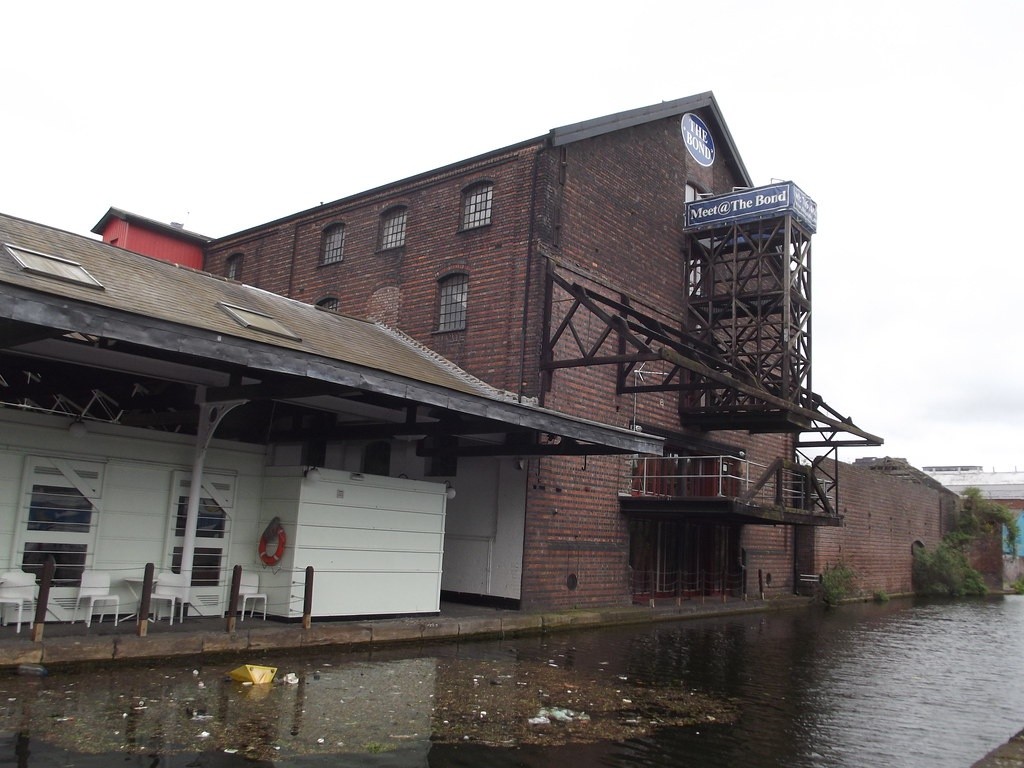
[304,465,321,481]
[68,412,86,440]
[350,472,364,481]
[444,480,457,500]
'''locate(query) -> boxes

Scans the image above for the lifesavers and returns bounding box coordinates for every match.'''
[258,525,285,564]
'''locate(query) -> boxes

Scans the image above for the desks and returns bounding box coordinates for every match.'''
[119,577,157,623]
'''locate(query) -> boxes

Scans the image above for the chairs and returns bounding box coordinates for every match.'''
[70,570,119,628]
[151,573,189,624]
[240,572,267,622]
[0,572,37,633]
